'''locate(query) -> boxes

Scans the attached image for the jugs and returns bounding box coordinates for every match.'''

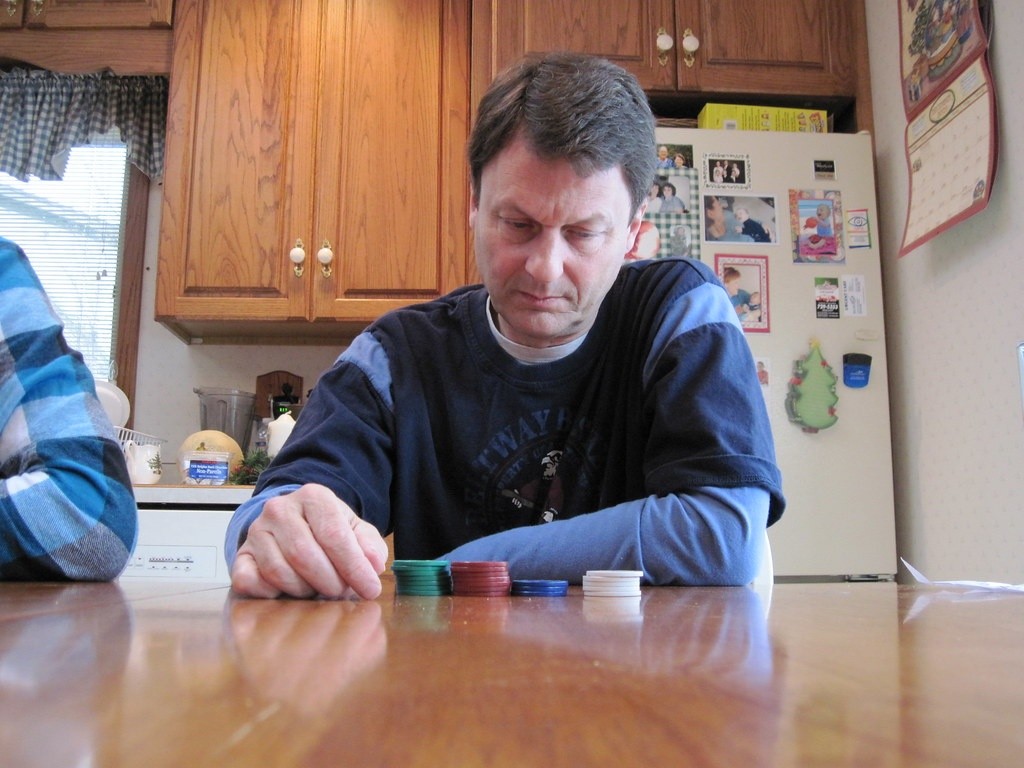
[125,440,162,484]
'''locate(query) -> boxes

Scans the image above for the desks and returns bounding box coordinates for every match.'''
[0,578,1024,768]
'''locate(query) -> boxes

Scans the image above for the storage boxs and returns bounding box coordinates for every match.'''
[178,450,236,486]
[697,101,834,133]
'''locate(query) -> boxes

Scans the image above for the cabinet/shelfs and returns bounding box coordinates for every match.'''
[0,0,174,76]
[491,0,856,118]
[153,0,491,347]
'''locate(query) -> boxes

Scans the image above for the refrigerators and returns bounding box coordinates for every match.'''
[620,127,899,584]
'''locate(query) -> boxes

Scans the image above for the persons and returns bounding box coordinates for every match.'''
[655,144,691,170]
[703,195,779,244]
[811,203,833,237]
[711,159,745,184]
[0,233,139,586]
[221,49,786,601]
[722,267,763,324]
[642,180,687,213]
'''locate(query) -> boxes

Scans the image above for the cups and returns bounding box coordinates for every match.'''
[192,386,262,457]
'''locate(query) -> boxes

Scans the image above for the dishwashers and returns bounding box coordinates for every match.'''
[113,507,235,577]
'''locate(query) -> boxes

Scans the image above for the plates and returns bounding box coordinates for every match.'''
[176,429,244,479]
[95,380,131,428]
